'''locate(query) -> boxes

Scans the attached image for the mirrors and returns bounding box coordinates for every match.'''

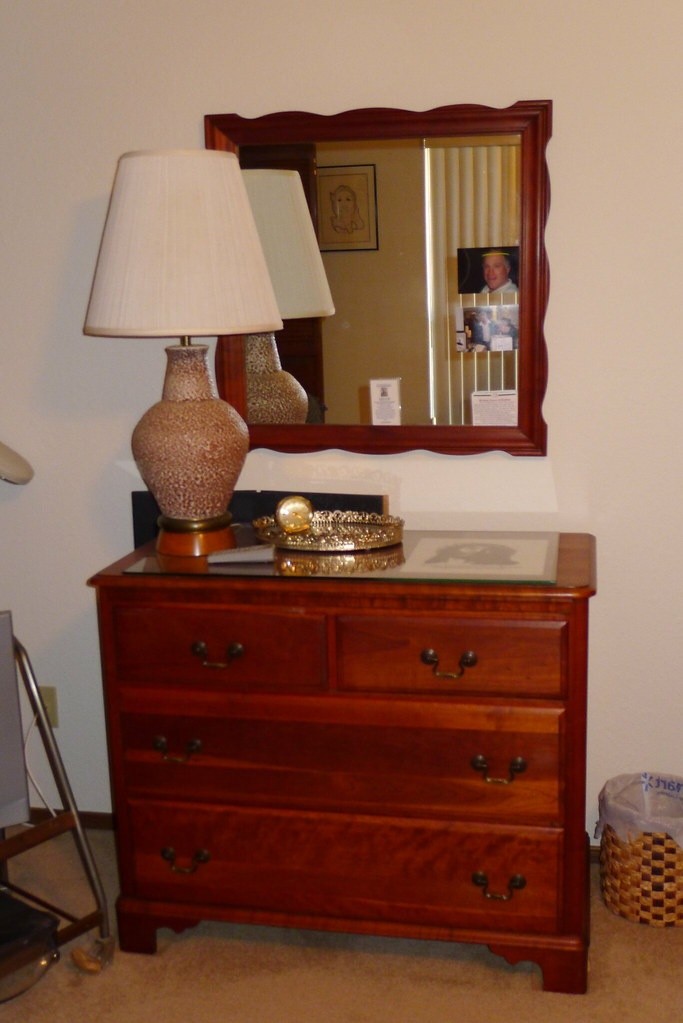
[202,97,554,458]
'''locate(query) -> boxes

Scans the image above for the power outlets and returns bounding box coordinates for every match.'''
[32,685,58,728]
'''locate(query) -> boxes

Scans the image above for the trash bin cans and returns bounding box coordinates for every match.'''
[600,768,683,925]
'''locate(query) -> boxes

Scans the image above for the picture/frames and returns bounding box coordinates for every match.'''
[318,163,378,252]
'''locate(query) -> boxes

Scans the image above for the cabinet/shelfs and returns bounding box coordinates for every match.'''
[86,530,596,996]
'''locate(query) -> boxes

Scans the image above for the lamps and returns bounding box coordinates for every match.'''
[239,167,338,425]
[82,147,285,578]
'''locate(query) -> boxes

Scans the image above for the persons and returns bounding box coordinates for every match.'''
[479,250,518,293]
[469,311,518,349]
